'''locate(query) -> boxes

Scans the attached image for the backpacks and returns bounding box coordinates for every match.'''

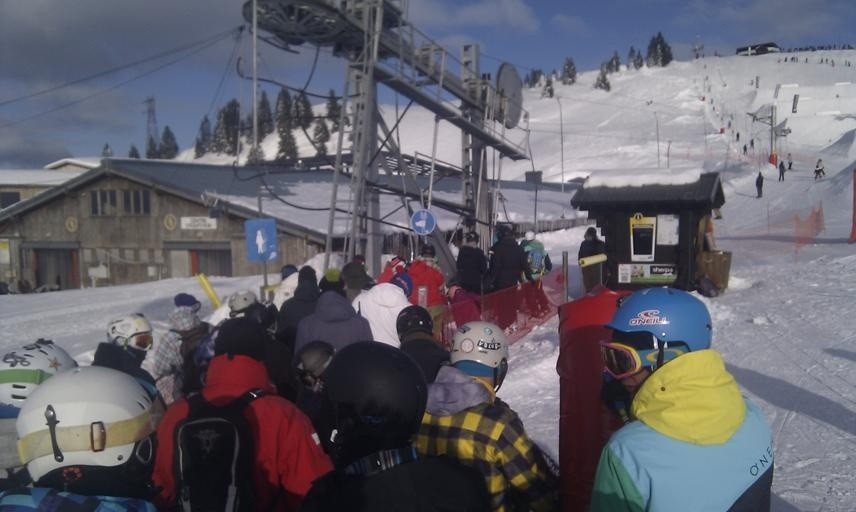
[168,321,210,372]
[171,389,281,512]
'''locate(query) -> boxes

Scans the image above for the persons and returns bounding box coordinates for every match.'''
[755,171,763,197]
[779,161,785,181]
[818,158,825,175]
[298,341,490,512]
[149,317,336,512]
[820,58,852,67]
[0,367,168,512]
[290,340,337,454]
[90,313,166,409]
[779,56,799,62]
[578,227,609,292]
[215,217,555,342]
[787,153,793,170]
[588,286,775,512]
[737,132,739,140]
[407,321,560,511]
[154,292,214,406]
[814,165,822,179]
[805,57,808,63]
[743,144,749,155]
[750,138,755,149]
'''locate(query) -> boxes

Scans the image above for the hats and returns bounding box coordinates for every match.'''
[214,317,269,364]
[353,256,365,264]
[319,268,345,290]
[299,265,316,281]
[392,257,406,275]
[526,231,535,240]
[175,293,201,313]
[283,265,297,280]
[585,228,596,241]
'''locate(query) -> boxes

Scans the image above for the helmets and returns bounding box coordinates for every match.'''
[106,312,153,351]
[449,320,510,369]
[604,287,713,352]
[298,340,337,380]
[422,244,435,257]
[15,366,168,483]
[466,231,479,241]
[325,340,429,447]
[391,272,413,297]
[495,222,514,239]
[229,291,257,318]
[396,305,434,341]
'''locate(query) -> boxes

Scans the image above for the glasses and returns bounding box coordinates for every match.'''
[598,338,690,380]
[16,378,167,466]
